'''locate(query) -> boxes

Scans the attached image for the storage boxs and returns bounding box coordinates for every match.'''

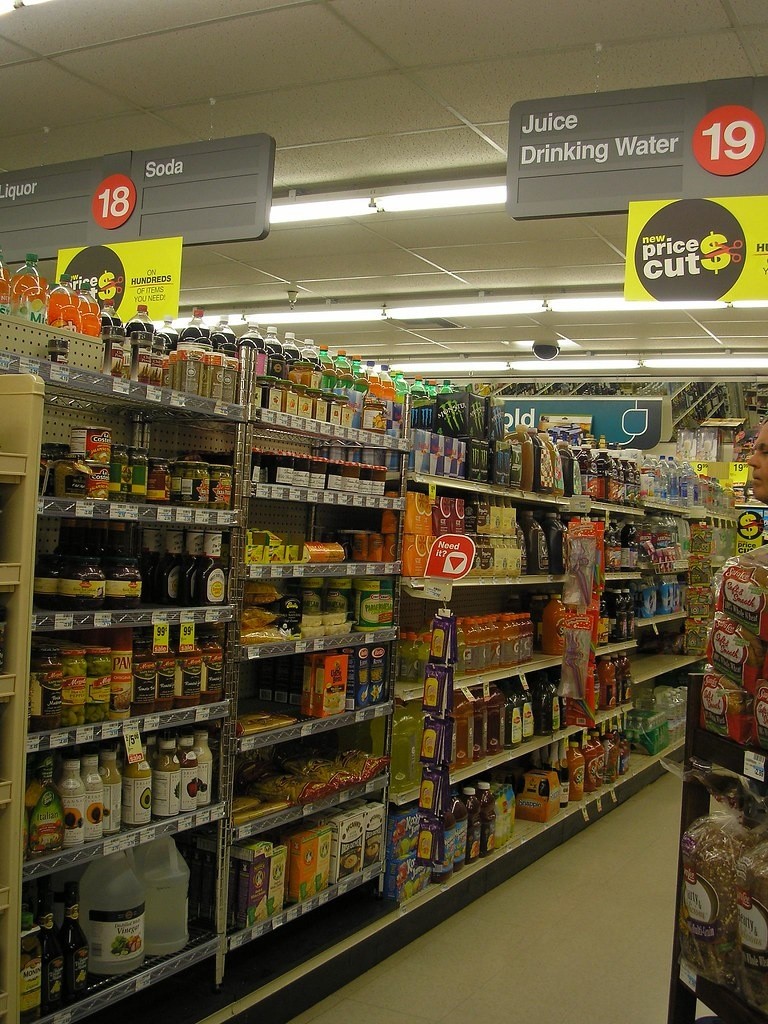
[184,643,387,927]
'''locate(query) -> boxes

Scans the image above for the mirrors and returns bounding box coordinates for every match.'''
[485,379,768,444]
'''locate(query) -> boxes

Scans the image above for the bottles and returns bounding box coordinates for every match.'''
[500,383,632,397]
[672,382,743,441]
[20,913,40,1023]
[37,875,63,1008]
[635,381,666,396]
[0,248,454,402]
[131,632,223,713]
[79,852,145,974]
[60,881,89,996]
[36,518,225,609]
[642,457,734,558]
[133,837,190,954]
[23,730,211,860]
[340,575,685,883]
[472,434,640,572]
[52,865,87,927]
[22,883,33,913]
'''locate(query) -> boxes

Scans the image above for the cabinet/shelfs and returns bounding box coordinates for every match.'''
[364,310,768,1024]
[222,349,412,980]
[2,311,252,1024]
[0,373,45,1024]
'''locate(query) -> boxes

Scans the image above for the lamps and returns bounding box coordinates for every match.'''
[150,173,768,329]
